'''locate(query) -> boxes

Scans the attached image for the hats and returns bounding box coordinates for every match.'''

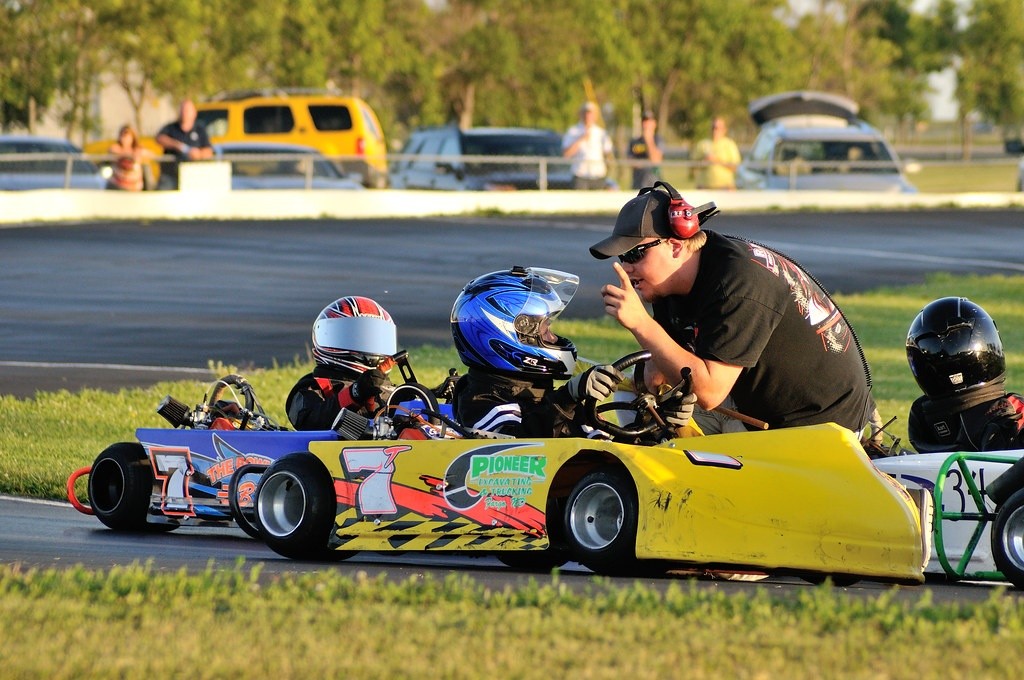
[589,192,671,260]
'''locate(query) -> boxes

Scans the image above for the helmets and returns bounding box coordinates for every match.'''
[450,266,577,381]
[905,297,1007,395]
[311,296,397,376]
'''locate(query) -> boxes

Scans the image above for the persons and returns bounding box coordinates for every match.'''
[627,109,664,189]
[905,296,1024,454]
[588,181,877,444]
[156,100,214,190]
[104,123,154,190]
[689,118,741,190]
[449,265,698,447]
[560,101,616,190]
[285,295,457,441]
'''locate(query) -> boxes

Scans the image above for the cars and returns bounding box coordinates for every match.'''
[105,139,373,193]
[730,87,919,195]
[0,134,110,192]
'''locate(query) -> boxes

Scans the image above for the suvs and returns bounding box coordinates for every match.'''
[80,89,390,182]
[389,126,616,194]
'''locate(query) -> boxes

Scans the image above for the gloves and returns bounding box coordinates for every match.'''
[659,385,697,427]
[567,364,625,405]
[348,369,391,402]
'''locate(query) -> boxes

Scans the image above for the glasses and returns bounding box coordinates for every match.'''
[617,240,668,264]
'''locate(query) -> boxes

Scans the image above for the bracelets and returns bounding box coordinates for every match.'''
[181,144,190,155]
